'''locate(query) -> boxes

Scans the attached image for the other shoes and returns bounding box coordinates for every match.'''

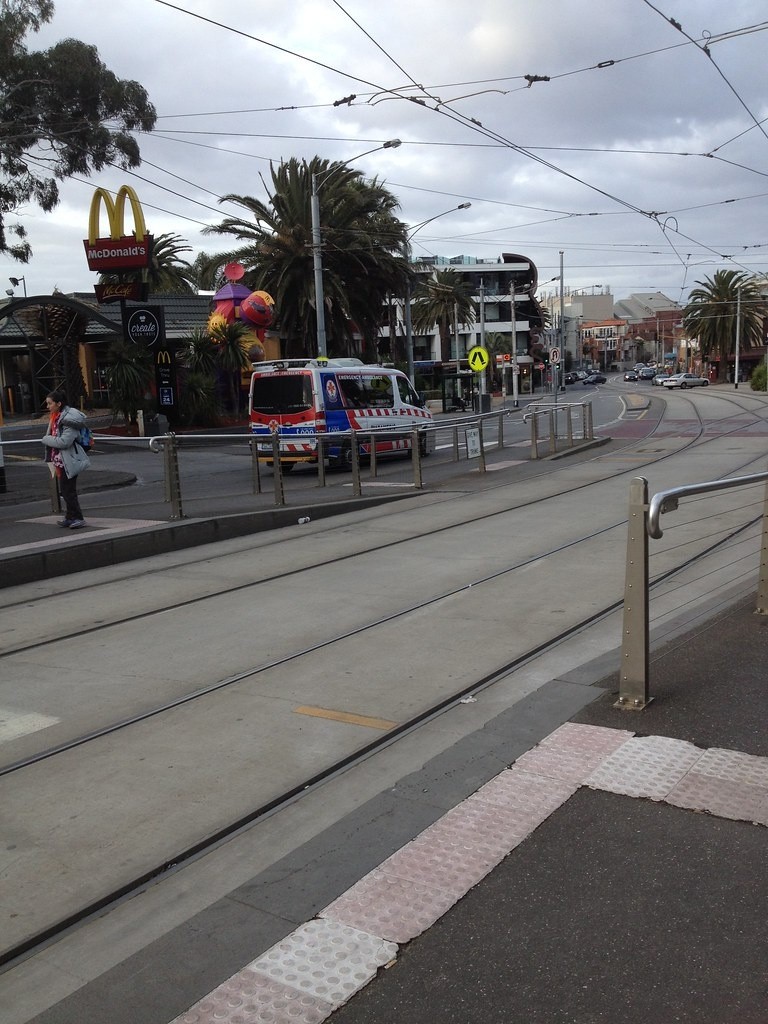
[69,518,87,528]
[56,519,73,527]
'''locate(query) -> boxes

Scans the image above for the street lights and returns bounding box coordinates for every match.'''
[403,202,473,389]
[478,277,531,394]
[310,139,403,360]
[551,283,604,390]
[509,276,561,407]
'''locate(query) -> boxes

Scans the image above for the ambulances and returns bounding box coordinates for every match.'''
[248,358,437,470]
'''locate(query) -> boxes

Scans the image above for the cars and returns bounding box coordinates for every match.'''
[565,369,601,384]
[652,374,672,386]
[624,371,638,382]
[582,375,607,386]
[663,373,711,389]
[632,358,672,380]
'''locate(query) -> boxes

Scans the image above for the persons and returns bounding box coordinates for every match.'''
[464,388,471,406]
[42,390,87,528]
[647,361,685,375]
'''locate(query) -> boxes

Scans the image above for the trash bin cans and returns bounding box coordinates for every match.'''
[473,393,491,414]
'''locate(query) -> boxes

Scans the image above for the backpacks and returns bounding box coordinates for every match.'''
[59,422,94,452]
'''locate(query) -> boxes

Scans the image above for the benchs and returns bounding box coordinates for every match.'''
[445,399,459,413]
